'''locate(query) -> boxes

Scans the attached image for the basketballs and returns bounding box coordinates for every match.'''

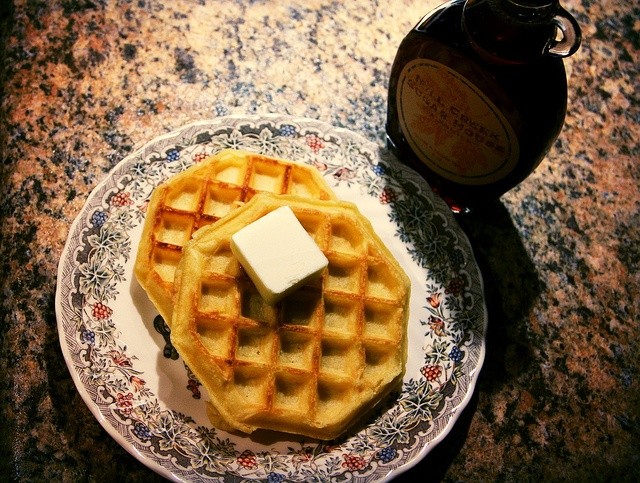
[173,193,412,442]
[133,149,339,329]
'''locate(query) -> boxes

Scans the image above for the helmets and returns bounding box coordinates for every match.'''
[56,114,488,483]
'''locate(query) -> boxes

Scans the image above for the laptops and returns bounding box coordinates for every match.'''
[228,204,329,304]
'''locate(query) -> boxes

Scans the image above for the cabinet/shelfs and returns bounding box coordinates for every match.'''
[385,0,582,216]
